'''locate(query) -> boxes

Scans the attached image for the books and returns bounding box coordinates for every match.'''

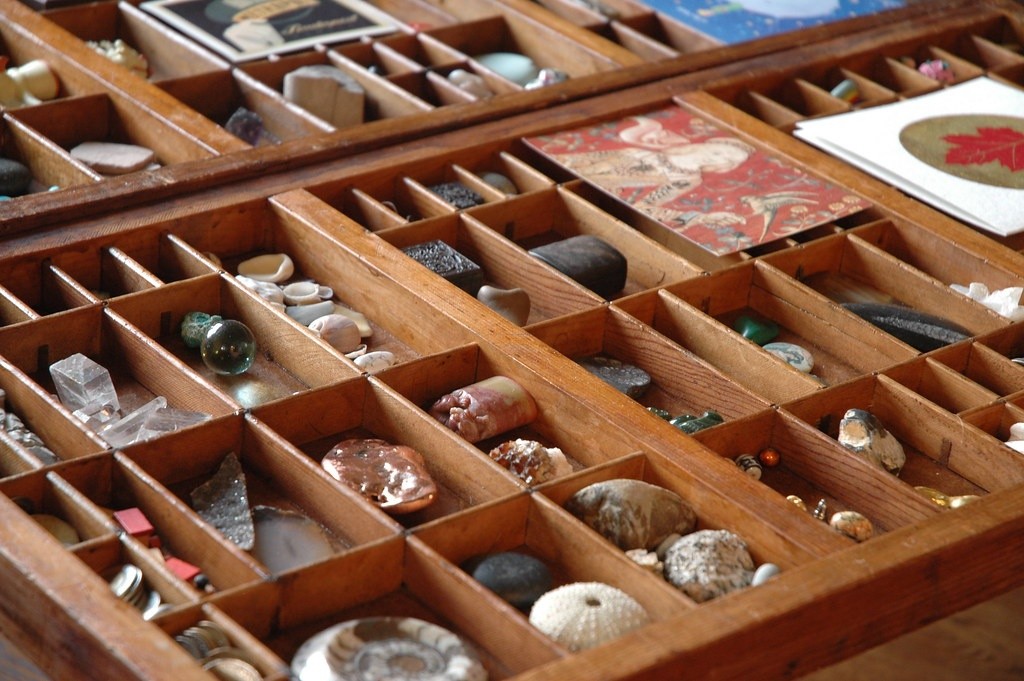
[793,77,1024,236]
[521,106,873,258]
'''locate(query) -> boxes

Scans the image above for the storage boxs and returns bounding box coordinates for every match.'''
[0,0,1010,238]
[2,6,1023,678]
